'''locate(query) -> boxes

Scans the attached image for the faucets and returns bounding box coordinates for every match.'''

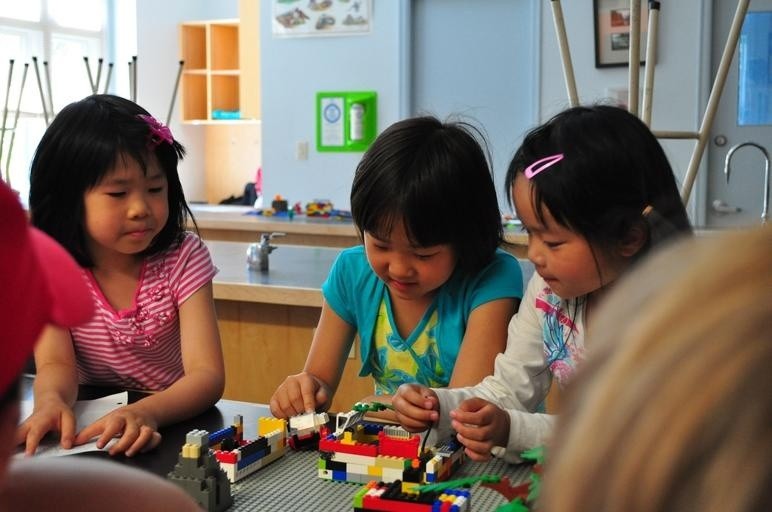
[724,141,771,226]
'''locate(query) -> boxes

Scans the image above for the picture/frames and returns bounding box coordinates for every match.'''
[593,0,655,72]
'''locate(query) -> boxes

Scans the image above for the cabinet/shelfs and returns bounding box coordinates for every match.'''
[180,21,261,124]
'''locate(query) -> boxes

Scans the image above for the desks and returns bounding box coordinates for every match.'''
[194,239,537,414]
[180,211,525,258]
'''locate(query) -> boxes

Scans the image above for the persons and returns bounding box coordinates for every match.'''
[392,105,692,462]
[269,117,523,424]
[532,225,772,511]
[0,180,203,512]
[15,95,225,458]
